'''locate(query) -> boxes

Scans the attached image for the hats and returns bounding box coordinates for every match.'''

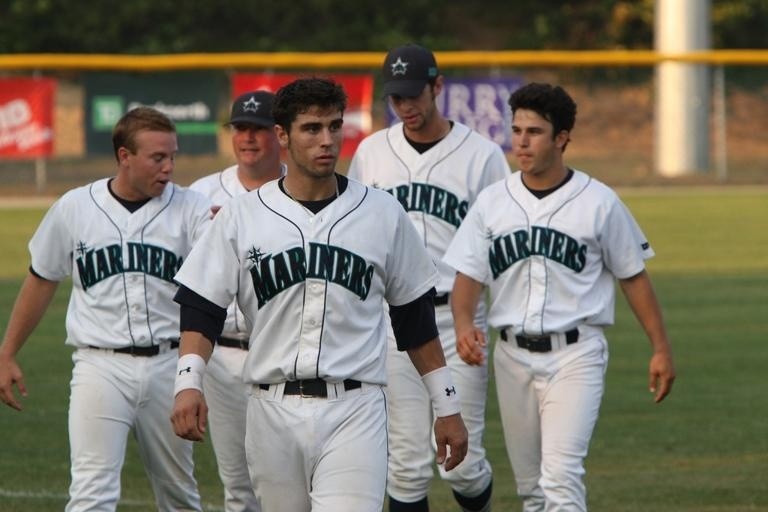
[223,91,276,128]
[381,43,437,98]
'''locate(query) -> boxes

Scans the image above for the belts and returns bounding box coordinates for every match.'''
[260,377,360,399]
[501,331,579,354]
[433,291,452,306]
[215,335,249,351]
[88,341,179,356]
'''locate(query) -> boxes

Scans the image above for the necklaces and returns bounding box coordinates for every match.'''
[281,171,339,219]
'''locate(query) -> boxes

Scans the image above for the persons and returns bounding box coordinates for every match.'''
[1,105,228,512]
[172,77,470,512]
[345,42,510,512]
[183,90,288,512]
[440,82,676,512]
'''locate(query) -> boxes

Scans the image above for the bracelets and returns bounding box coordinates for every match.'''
[174,354,207,396]
[421,365,463,419]
